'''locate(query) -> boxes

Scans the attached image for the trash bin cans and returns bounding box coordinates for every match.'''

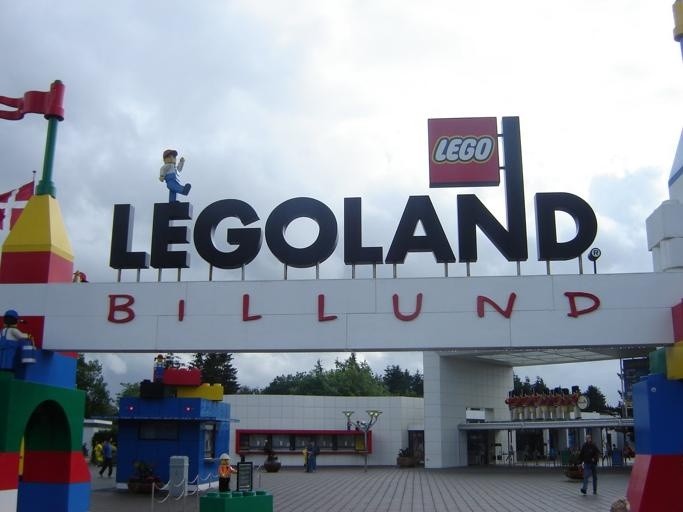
[306,451,316,472]
[612,450,622,469]
[169,456,189,497]
[561,449,570,468]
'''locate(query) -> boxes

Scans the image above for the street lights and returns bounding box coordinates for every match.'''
[341,409,383,472]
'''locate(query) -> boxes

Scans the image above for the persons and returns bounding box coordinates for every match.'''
[83,434,117,478]
[576,434,601,494]
[623,442,632,463]
[302,439,320,473]
